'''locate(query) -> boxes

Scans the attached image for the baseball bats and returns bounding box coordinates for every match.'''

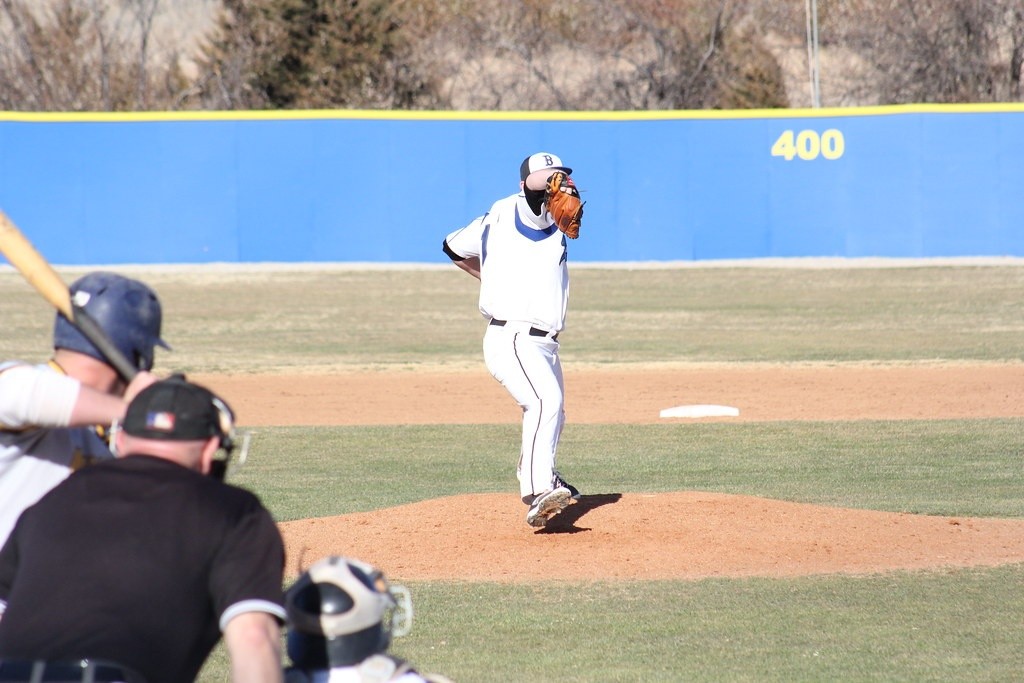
[0,209,140,387]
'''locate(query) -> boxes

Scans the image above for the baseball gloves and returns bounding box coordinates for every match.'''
[544,171,586,240]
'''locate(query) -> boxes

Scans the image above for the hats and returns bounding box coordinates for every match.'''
[520,152,572,183]
[123,376,235,441]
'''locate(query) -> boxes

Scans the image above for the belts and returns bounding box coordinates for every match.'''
[0,661,123,683]
[491,319,557,340]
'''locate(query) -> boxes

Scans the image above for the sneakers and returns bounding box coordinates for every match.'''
[552,470,581,500]
[526,485,572,527]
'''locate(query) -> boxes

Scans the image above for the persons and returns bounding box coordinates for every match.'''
[0,270,173,616]
[278,555,455,683]
[0,374,289,683]
[442,151,588,525]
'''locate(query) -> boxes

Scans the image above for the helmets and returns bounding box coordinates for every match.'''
[52,271,170,385]
[286,561,378,671]
